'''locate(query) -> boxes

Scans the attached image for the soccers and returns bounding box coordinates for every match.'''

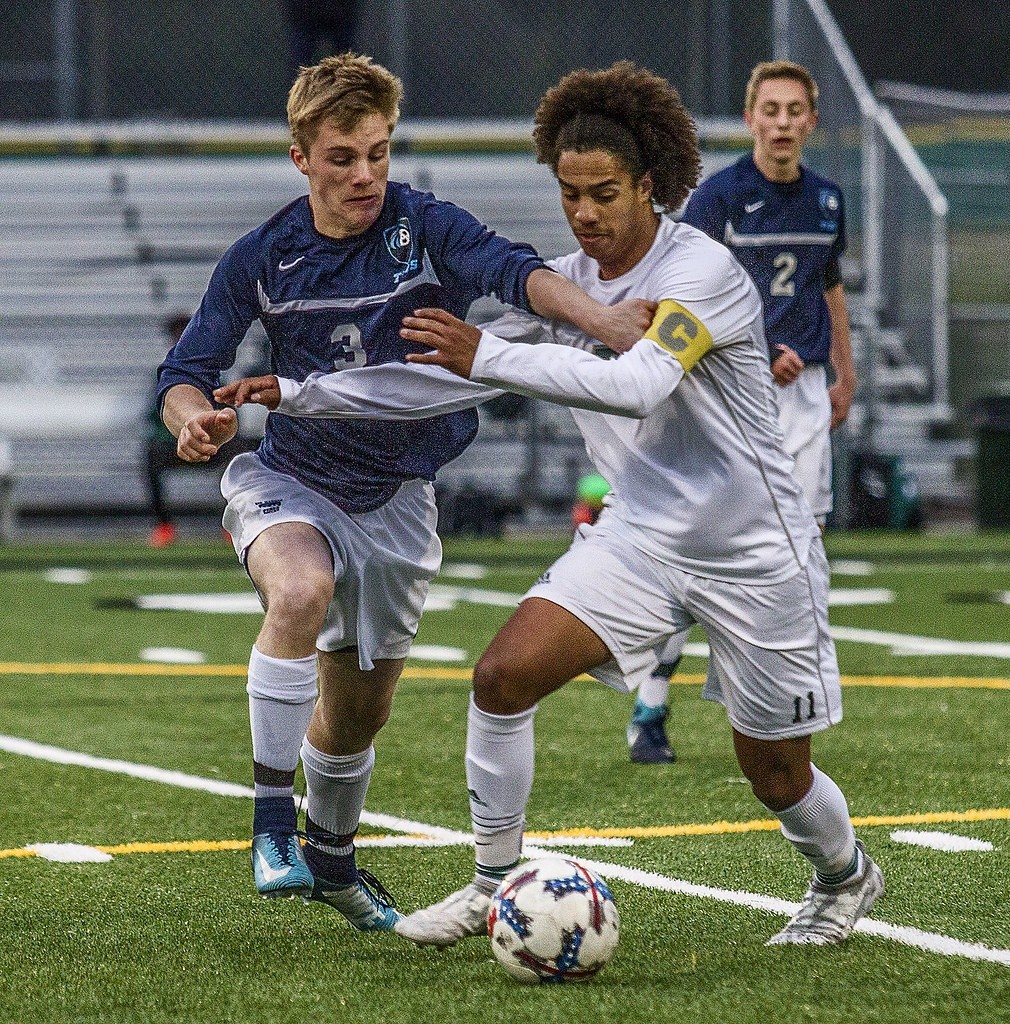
[484,857,622,983]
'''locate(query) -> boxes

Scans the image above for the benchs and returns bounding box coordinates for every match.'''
[0,111,976,545]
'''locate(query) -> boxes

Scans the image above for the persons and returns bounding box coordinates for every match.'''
[211,59,885,962]
[142,313,236,547]
[160,51,657,930]
[628,61,858,763]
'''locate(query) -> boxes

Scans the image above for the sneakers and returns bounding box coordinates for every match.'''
[764,839,884,947]
[394,887,491,951]
[299,845,408,932]
[249,827,336,900]
[626,721,676,763]
